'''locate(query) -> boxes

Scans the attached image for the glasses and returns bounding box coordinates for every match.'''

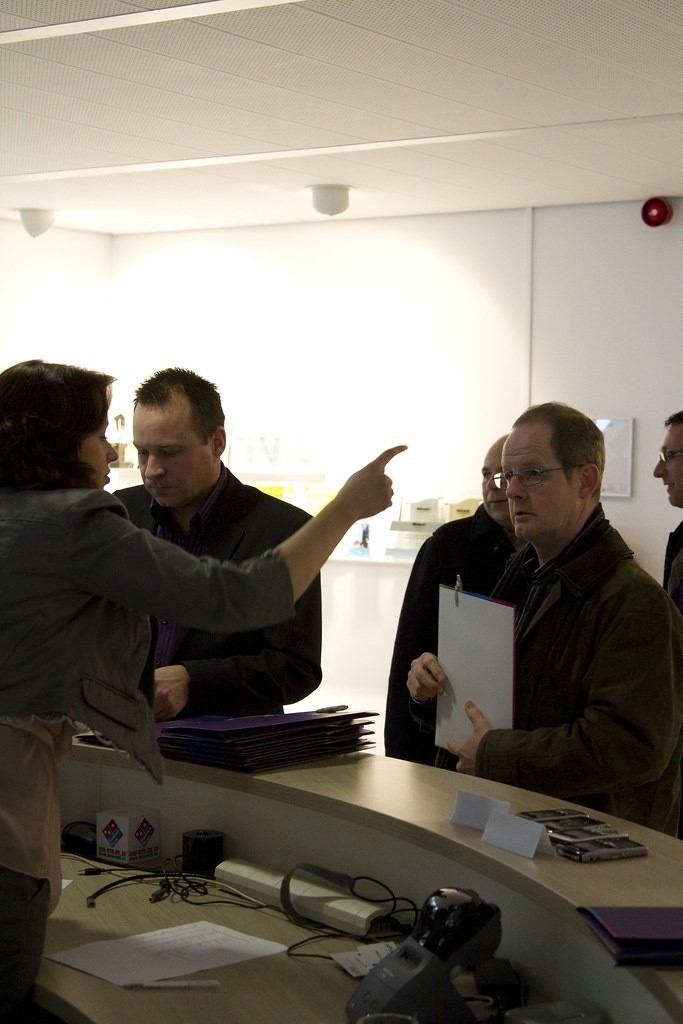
[657,449,683,464]
[492,463,586,490]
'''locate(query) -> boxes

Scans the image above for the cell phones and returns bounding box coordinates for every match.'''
[514,809,647,864]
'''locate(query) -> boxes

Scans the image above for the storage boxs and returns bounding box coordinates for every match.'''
[95,804,161,864]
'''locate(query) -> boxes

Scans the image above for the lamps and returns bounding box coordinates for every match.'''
[302,183,356,218]
[14,208,57,239]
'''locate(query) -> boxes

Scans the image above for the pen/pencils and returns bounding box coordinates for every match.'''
[315,705,348,713]
[454,574,462,605]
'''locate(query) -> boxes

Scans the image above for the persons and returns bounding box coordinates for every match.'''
[113,368,322,721]
[0,361,406,1024]
[406,403,683,839]
[384,435,528,767]
[654,409,683,617]
